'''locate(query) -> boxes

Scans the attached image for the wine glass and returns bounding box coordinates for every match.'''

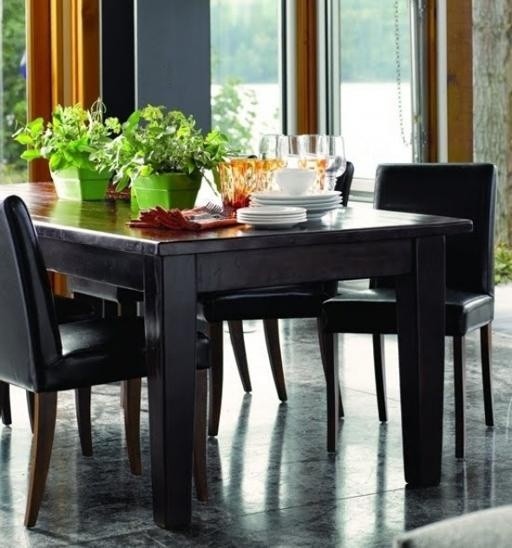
[260,133,347,192]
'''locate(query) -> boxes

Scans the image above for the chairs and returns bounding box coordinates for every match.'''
[322,165,498,458]
[2,193,213,529]
[206,163,356,436]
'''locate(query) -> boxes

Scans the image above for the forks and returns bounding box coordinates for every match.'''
[182,202,222,222]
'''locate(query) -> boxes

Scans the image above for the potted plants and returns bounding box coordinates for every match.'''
[10,96,243,211]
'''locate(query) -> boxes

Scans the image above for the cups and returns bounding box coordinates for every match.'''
[275,168,318,194]
[218,157,328,219]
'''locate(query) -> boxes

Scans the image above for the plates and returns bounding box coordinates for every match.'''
[236,192,343,230]
